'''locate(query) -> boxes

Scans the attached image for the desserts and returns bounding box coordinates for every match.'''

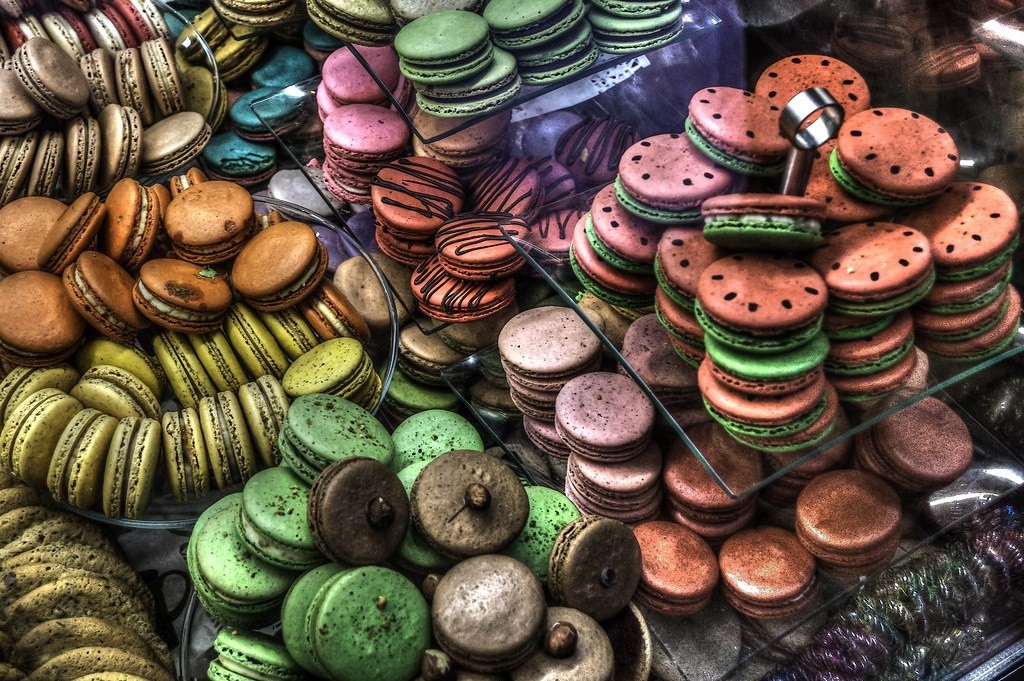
[0,0,1024,681]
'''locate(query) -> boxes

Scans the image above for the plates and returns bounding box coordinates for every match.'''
[59,196,399,530]
[100,0,221,200]
[182,458,556,681]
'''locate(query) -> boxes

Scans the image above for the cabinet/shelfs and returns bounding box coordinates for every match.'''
[251,0,1024,681]
